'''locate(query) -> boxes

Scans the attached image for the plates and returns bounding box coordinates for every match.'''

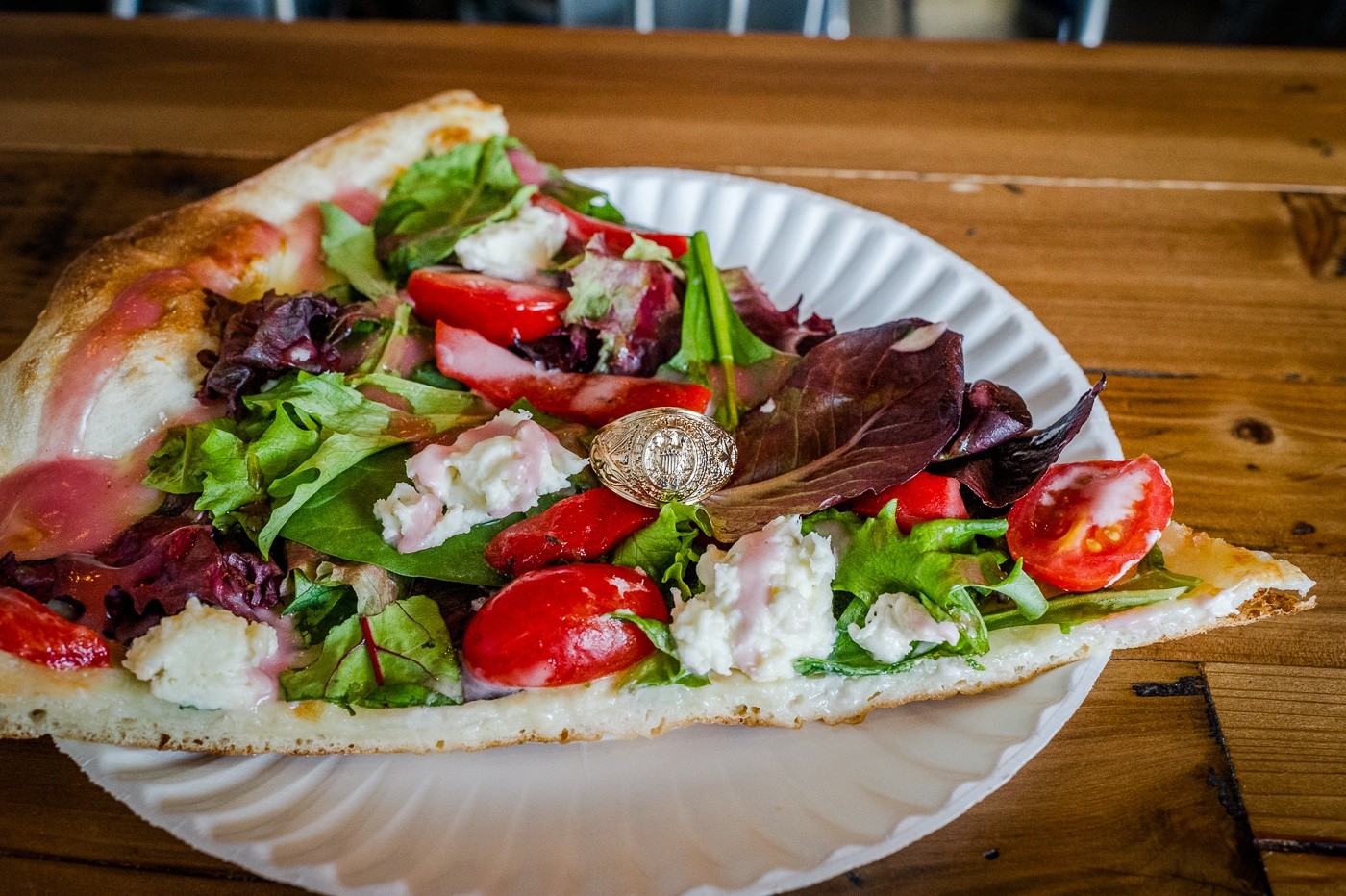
[1,168,1126,896]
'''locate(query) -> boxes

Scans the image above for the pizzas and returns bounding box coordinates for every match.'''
[0,86,1319,755]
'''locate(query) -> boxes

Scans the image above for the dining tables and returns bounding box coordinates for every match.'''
[0,19,1346,896]
